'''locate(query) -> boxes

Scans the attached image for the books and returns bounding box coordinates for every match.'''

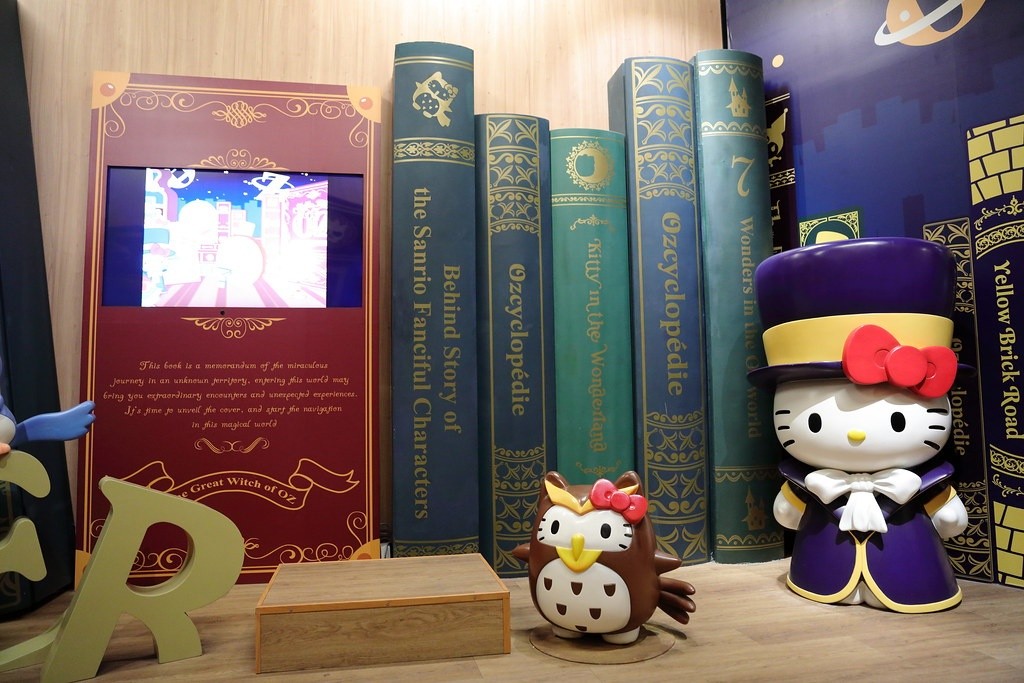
[388,42,785,578]
